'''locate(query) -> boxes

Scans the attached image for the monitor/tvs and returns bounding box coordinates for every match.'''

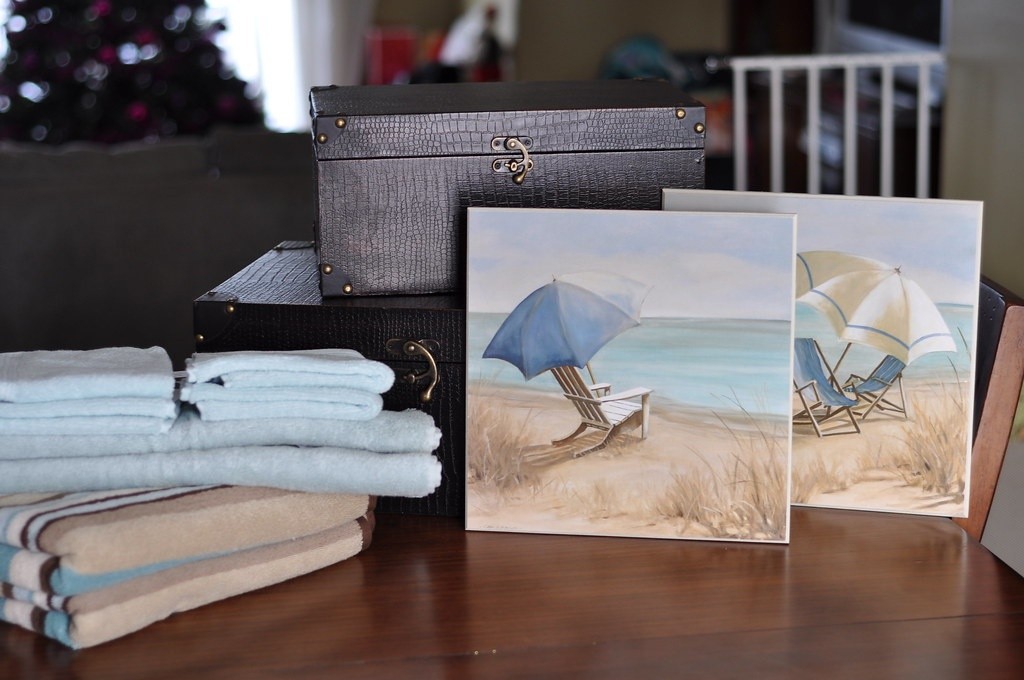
[831,0,950,87]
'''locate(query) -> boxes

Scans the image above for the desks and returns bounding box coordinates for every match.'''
[0,505,1024,680]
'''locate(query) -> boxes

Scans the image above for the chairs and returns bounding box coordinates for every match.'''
[0,124,314,371]
[729,48,947,199]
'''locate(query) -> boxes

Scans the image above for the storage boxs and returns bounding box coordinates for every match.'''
[190,238,464,508]
[305,73,710,308]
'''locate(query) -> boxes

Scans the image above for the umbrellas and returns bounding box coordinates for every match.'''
[483,272,659,381]
[795,250,958,366]
